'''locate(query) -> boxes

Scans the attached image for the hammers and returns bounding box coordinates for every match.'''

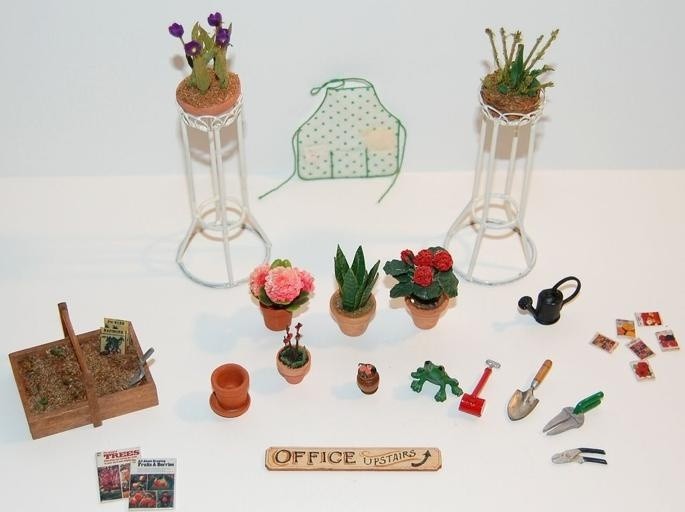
[458,359,501,418]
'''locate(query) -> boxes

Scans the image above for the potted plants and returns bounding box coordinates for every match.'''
[329,244,381,336]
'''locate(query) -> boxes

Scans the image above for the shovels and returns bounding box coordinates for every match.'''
[508,360,552,421]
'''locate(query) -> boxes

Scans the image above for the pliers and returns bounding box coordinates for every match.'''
[543,392,604,435]
[551,448,607,465]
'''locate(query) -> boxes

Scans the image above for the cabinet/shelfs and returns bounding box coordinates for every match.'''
[442,96,546,286]
[174,102,271,290]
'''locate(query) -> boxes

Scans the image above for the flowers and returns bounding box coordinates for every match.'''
[483,27,559,96]
[247,260,315,307]
[358,363,376,378]
[383,247,459,302]
[168,12,232,95]
[280,323,307,367]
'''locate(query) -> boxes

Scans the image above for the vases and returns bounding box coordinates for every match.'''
[404,293,449,330]
[177,70,241,116]
[211,363,249,411]
[277,344,311,384]
[357,373,379,392]
[481,72,541,121]
[260,301,293,331]
